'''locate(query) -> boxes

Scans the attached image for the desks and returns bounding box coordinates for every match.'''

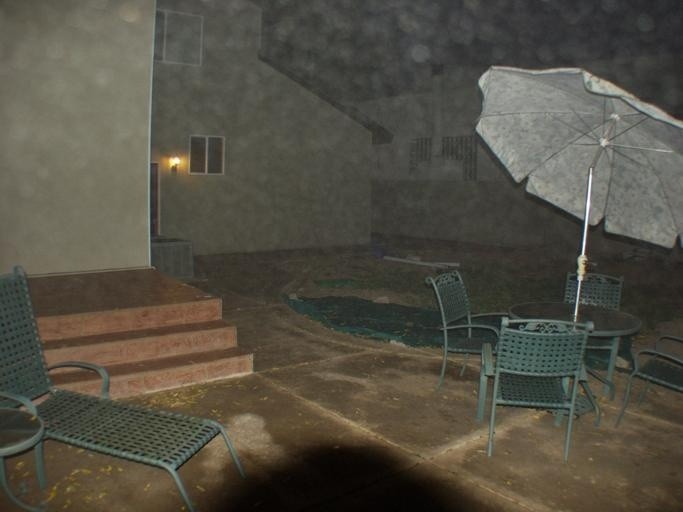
[508,299,643,397]
[0,405,48,511]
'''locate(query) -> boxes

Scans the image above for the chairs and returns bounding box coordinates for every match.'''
[426,269,508,393]
[0,267,248,512]
[568,273,624,310]
[478,316,595,459]
[612,336,682,431]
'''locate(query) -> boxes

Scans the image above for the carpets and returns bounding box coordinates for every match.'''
[283,290,635,374]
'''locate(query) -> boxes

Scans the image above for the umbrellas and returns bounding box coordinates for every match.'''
[474,62,683,316]
